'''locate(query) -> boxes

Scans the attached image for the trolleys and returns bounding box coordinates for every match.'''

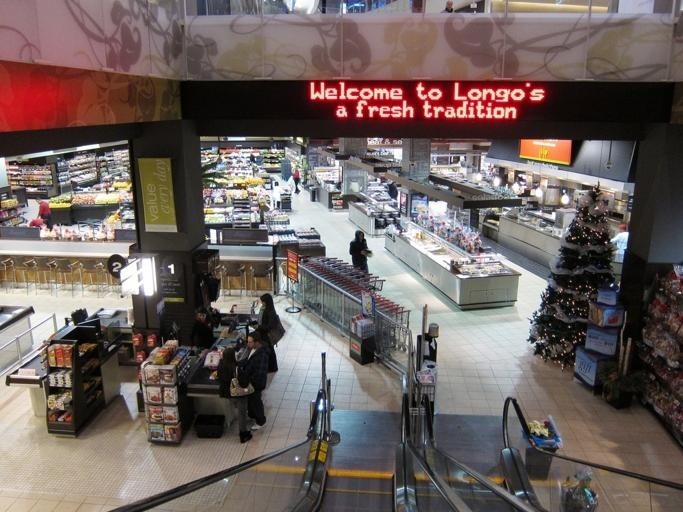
[303,255,412,352]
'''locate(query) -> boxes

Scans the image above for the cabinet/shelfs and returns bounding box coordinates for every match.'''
[7,152,107,198]
[46,316,104,435]
[631,273,683,450]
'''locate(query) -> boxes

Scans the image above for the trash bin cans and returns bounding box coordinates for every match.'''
[525,418,561,481]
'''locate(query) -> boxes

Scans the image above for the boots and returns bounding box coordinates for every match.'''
[239,431,252,443]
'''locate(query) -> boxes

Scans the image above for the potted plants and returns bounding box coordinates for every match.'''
[599,359,642,410]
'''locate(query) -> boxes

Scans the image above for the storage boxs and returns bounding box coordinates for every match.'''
[572,282,624,391]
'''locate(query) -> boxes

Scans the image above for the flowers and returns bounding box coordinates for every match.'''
[522,417,558,448]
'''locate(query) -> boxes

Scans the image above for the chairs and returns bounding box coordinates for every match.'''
[221,260,274,297]
[0,254,108,299]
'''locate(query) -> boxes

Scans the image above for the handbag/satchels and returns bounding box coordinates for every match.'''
[229,366,255,396]
[264,315,287,347]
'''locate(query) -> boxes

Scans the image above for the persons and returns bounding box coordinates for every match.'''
[28,218,50,227]
[250,153,256,162]
[194,306,215,349]
[242,331,269,430]
[218,348,253,444]
[292,165,301,194]
[258,292,285,372]
[387,184,398,202]
[349,230,373,274]
[36,196,53,229]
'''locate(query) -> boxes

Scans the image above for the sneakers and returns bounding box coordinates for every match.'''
[251,423,265,429]
